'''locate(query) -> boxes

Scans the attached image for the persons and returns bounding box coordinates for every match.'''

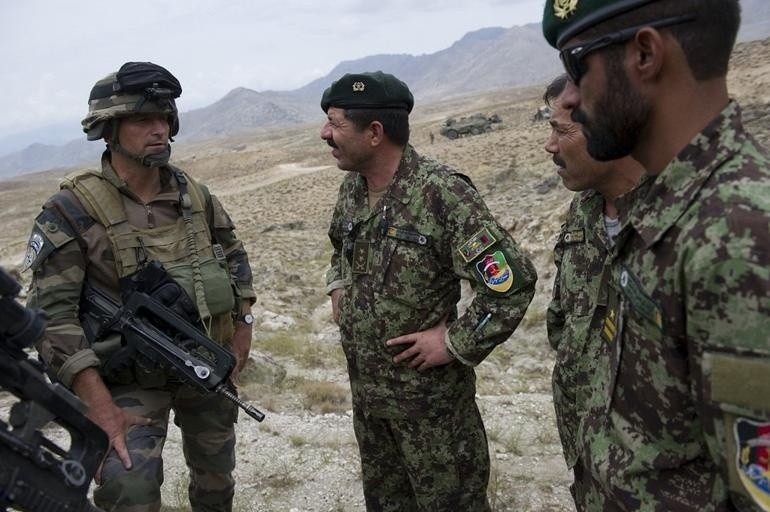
[26,59,256,511]
[315,67,540,511]
[543,2,770,512]
[543,74,653,509]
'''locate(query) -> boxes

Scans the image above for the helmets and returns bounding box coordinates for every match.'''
[80,61,182,142]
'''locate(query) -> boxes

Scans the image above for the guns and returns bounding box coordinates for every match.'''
[1,266,112,511]
[78,279,265,422]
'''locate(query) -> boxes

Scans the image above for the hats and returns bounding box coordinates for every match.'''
[541,2,729,51]
[320,70,415,115]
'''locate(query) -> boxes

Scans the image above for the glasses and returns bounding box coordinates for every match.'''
[559,15,686,88]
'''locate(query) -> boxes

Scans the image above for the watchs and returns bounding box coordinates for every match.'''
[235,313,254,324]
